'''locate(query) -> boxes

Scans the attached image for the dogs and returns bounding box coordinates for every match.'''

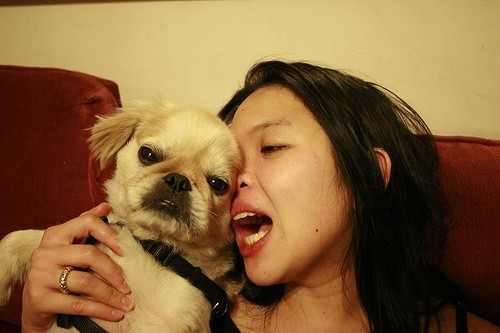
[0,88,244,333]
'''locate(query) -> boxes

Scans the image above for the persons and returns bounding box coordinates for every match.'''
[11,61,500,333]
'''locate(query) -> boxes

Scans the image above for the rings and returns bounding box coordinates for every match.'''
[59,266,74,294]
